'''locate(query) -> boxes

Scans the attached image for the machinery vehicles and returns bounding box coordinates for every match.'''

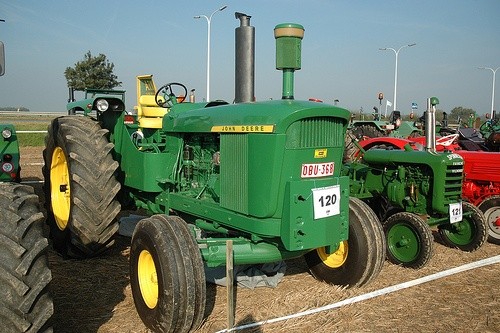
[0,11,500,333]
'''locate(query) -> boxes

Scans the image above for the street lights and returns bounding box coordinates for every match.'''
[194,4,227,103]
